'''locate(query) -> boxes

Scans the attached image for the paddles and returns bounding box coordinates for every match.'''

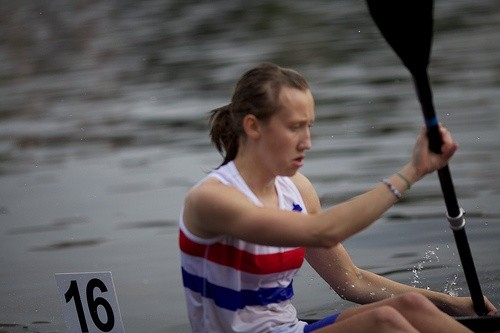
[366,0,490,318]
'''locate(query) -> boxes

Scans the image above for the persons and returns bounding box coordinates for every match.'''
[178,62,496,333]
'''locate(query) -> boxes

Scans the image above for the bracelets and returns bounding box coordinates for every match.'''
[397,172,411,189]
[381,178,404,200]
